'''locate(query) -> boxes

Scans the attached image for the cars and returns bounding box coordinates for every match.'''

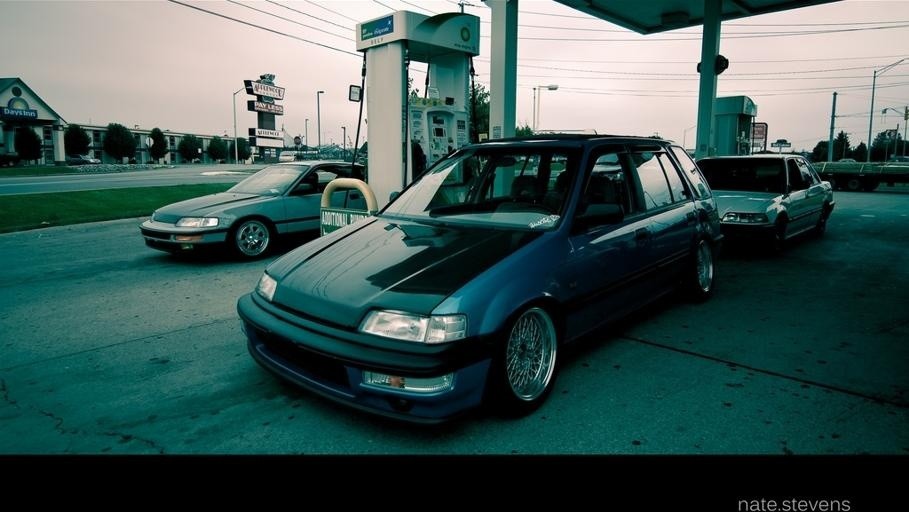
[237,133,725,427]
[695,154,834,247]
[840,159,856,162]
[139,158,367,262]
[67,154,101,166]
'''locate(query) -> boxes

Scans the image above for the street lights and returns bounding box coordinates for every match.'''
[232,86,253,166]
[883,106,907,160]
[304,118,309,158]
[341,126,346,159]
[536,83,559,131]
[317,90,325,152]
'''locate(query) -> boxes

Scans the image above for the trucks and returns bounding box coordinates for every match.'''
[809,161,909,191]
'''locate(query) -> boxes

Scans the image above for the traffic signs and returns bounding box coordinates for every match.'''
[772,143,791,147]
